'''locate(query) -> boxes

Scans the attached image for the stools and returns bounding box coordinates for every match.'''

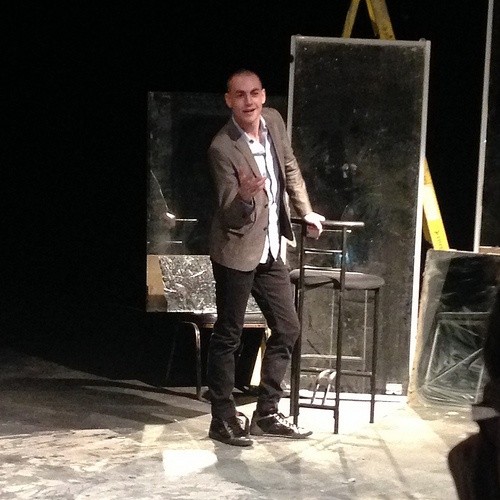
[154,298,271,406]
[283,210,385,435]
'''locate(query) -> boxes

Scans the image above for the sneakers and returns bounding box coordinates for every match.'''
[208,411,312,447]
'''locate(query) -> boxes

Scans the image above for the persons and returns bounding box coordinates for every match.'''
[207,69,314,447]
